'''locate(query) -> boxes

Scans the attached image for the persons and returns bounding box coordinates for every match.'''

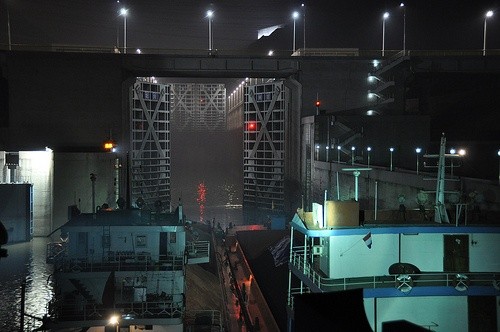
[192,230,199,243]
[217,223,224,234]
[398,195,406,212]
[415,189,428,215]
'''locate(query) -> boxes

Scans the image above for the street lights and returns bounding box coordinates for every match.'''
[206,10,213,51]
[292,10,301,53]
[301,4,307,56]
[483,11,493,57]
[338,146,341,164]
[326,146,329,162]
[367,147,371,168]
[316,145,319,161]
[120,8,127,54]
[400,3,406,57]
[381,12,390,57]
[416,148,421,175]
[350,146,356,167]
[389,147,394,172]
[449,149,455,179]
[110,315,119,332]
